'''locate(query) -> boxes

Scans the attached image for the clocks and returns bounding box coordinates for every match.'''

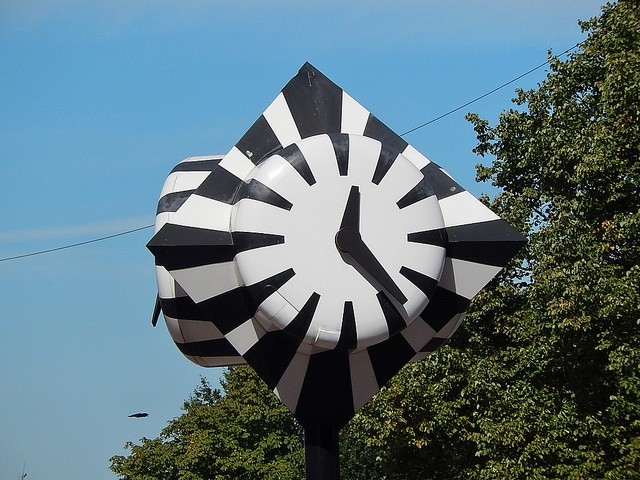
[146,62,529,441]
